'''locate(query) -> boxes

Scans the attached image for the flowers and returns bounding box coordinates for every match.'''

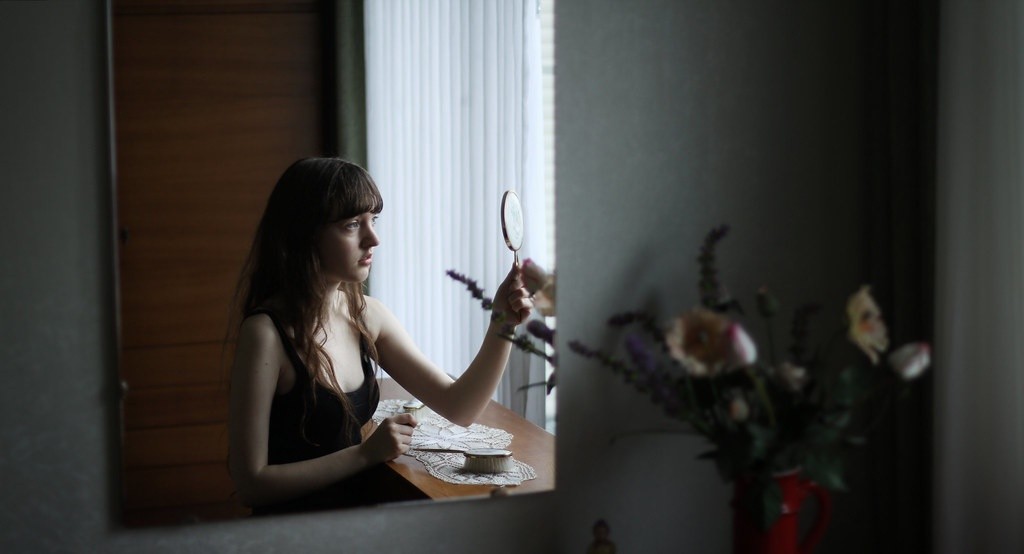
[566,223,930,494]
[444,270,559,395]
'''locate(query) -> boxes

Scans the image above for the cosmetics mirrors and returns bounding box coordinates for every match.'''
[501,190,523,266]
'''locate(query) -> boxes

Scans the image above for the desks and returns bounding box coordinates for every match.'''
[359,377,558,500]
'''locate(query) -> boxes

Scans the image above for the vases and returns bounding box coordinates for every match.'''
[731,472,832,553]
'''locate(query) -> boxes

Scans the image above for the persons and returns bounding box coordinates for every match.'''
[221,158,533,516]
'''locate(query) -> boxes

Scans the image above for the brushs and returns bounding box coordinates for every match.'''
[403,398,430,422]
[412,448,513,472]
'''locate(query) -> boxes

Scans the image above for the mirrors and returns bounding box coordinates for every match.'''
[101,0,560,529]
